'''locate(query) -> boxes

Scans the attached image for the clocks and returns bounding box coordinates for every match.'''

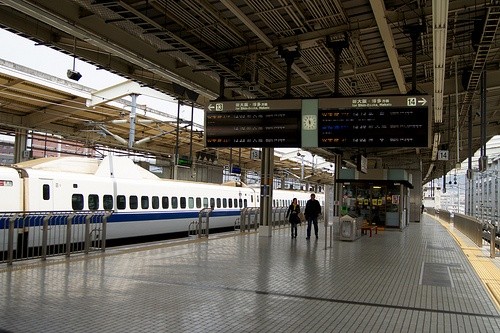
[303,115,318,130]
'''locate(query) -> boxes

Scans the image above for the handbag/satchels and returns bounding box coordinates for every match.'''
[295,216,302,224]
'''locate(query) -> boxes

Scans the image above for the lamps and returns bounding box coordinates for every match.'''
[67,55,82,82]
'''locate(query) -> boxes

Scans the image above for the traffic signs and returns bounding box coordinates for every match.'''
[204,98,301,149]
[316,97,429,148]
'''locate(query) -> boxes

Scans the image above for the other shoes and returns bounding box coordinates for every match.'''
[316,235,318,239]
[306,237,310,239]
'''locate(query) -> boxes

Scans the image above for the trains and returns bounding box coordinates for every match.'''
[0,155,325,255]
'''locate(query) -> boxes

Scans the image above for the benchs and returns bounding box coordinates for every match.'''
[360,223,380,237]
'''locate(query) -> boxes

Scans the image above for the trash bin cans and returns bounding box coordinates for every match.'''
[341,210,361,241]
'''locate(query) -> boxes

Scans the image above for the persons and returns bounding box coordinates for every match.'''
[304,194,321,239]
[286,198,301,237]
